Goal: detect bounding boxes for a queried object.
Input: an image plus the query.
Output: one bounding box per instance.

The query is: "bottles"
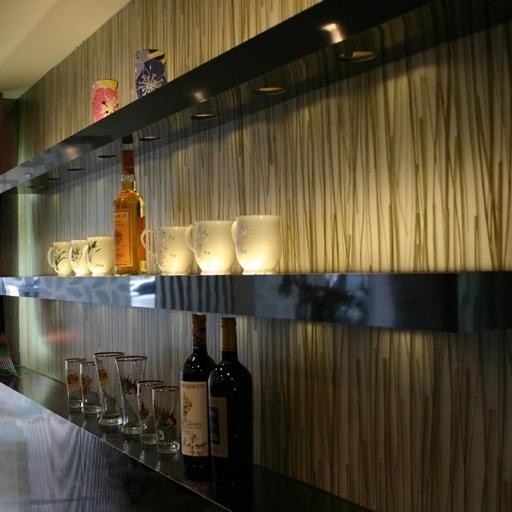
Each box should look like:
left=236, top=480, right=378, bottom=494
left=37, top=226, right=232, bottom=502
left=179, top=309, right=256, bottom=478
left=110, top=133, right=148, bottom=276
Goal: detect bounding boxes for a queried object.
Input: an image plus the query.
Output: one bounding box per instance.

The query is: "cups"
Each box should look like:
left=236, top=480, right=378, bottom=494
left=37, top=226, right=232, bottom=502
left=62, top=349, right=178, bottom=457
left=139, top=215, right=286, bottom=276
left=90, top=47, right=168, bottom=127
left=45, top=235, right=114, bottom=279
